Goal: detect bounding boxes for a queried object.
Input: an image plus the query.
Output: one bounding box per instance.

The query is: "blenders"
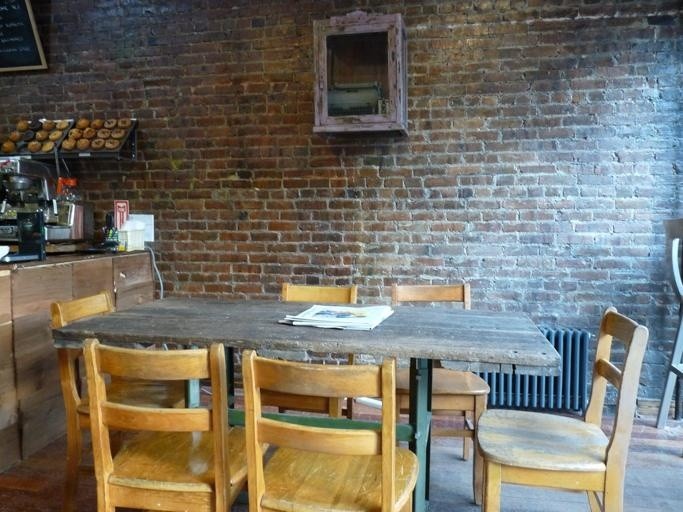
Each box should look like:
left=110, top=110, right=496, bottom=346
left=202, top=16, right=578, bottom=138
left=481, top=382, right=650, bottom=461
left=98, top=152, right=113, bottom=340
left=44, top=202, right=96, bottom=243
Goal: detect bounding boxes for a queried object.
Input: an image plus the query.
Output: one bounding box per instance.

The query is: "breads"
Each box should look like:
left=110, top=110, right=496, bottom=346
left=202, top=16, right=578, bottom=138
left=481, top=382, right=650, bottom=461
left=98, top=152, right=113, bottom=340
left=2, top=117, right=132, bottom=153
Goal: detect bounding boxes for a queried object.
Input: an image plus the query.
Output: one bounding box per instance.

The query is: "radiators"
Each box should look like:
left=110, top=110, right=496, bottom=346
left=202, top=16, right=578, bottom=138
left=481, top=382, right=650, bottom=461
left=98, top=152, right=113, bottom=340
left=476, top=324, right=590, bottom=413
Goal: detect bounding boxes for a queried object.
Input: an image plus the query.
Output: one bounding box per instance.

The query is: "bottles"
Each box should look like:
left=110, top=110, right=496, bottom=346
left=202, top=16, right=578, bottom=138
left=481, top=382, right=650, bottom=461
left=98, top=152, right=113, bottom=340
left=103, top=211, right=115, bottom=244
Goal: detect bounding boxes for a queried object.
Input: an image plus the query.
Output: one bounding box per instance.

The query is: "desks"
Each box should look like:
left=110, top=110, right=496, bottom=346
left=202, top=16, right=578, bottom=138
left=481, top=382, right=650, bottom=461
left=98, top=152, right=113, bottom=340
left=51, top=294, right=562, bottom=511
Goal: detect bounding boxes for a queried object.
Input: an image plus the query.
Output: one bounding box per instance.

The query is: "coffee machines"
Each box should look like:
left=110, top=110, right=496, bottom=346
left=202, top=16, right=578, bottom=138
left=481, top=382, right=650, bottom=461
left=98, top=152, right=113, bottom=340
left=0, top=157, right=54, bottom=262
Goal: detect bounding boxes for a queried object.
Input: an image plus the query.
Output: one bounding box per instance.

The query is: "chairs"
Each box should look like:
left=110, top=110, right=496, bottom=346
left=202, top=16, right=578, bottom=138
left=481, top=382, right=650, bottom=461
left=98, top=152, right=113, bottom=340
left=82, top=336, right=270, bottom=511
left=50, top=291, right=187, bottom=511
left=476, top=305, right=650, bottom=512
left=391, top=282, right=490, bottom=508
left=259, top=281, right=360, bottom=420
left=239, top=346, right=419, bottom=511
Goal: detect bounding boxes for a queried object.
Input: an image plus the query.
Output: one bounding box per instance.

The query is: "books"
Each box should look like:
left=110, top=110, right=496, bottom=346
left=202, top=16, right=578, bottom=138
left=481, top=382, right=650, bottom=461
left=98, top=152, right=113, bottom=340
left=277, top=304, right=395, bottom=331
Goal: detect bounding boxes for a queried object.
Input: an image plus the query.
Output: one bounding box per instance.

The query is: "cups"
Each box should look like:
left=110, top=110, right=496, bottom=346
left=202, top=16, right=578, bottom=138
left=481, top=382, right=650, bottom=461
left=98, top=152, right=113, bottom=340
left=114, top=230, right=127, bottom=253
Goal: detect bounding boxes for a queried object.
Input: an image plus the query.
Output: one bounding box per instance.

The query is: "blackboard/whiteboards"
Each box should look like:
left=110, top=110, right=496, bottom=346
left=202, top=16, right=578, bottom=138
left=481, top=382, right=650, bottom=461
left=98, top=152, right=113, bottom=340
left=0, top=0, right=49, bottom=72
